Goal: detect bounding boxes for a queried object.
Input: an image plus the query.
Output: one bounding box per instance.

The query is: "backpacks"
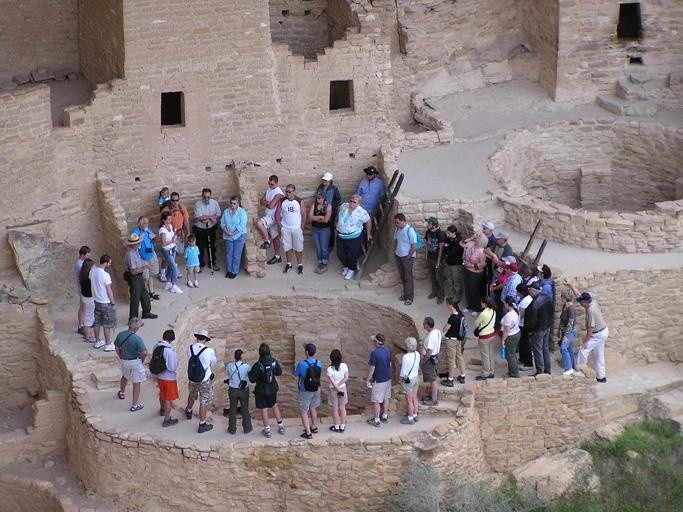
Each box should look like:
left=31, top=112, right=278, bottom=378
left=188, top=344, right=211, bottom=382
left=149, top=346, right=170, bottom=374
left=302, top=360, right=321, bottom=391
left=257, top=359, right=277, bottom=384
left=459, top=317, right=469, bottom=339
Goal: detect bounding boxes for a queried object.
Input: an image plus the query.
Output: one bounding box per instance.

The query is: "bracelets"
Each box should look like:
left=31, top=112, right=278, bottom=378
left=111, top=304, right=115, bottom=306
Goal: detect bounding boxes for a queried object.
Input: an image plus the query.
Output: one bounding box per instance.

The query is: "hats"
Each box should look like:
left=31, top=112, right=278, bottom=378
left=364, top=166, right=379, bottom=175
left=425, top=217, right=438, bottom=225
left=502, top=256, right=591, bottom=302
left=371, top=334, right=385, bottom=345
left=321, top=172, right=333, bottom=181
left=194, top=330, right=211, bottom=341
left=313, top=191, right=327, bottom=198
left=482, top=222, right=494, bottom=230
left=302, top=343, right=316, bottom=353
left=124, top=233, right=144, bottom=245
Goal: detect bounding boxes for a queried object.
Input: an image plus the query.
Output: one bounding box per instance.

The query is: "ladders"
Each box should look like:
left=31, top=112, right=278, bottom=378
left=355, top=169, right=405, bottom=281
left=517, top=219, right=548, bottom=283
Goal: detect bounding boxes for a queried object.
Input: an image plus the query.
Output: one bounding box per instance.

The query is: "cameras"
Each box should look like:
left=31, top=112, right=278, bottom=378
left=224, top=378, right=229, bottom=385
left=239, top=380, right=247, bottom=388
left=404, top=376, right=410, bottom=383
left=146, top=248, right=152, bottom=253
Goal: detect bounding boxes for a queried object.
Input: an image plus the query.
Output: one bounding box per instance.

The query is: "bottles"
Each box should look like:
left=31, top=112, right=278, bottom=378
left=499, top=346, right=505, bottom=359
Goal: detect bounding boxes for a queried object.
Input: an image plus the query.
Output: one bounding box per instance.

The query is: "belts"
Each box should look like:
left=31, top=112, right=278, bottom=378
left=592, top=325, right=606, bottom=333
left=123, top=358, right=140, bottom=360
left=446, top=337, right=457, bottom=340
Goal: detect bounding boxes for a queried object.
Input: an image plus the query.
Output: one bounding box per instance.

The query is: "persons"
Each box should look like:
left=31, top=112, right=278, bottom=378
left=399, top=337, right=422, bottom=424
left=132, top=215, right=160, bottom=300
left=153, top=329, right=180, bottom=427
left=92, top=255, right=116, bottom=352
left=423, top=217, right=610, bottom=387
left=114, top=318, right=148, bottom=412
left=248, top=343, right=285, bottom=437
left=159, top=186, right=247, bottom=294
left=227, top=349, right=254, bottom=434
left=421, top=317, right=442, bottom=406
left=76, top=246, right=91, bottom=334
left=257, top=166, right=384, bottom=280
left=327, top=350, right=348, bottom=433
left=366, top=333, right=391, bottom=427
left=186, top=330, right=217, bottom=433
left=79, top=259, right=96, bottom=343
left=294, top=344, right=322, bottom=439
left=393, top=213, right=417, bottom=305
left=125, top=232, right=158, bottom=327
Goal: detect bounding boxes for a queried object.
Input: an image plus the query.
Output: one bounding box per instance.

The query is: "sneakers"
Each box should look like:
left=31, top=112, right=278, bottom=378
left=261, top=240, right=355, bottom=280
left=399, top=293, right=478, bottom=316
left=148, top=263, right=236, bottom=301
left=78, top=312, right=157, bottom=351
left=160, top=406, right=344, bottom=438
left=441, top=359, right=606, bottom=387
left=368, top=397, right=438, bottom=427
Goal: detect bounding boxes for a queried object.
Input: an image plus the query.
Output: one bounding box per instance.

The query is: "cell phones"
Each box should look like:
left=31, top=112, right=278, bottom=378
left=337, top=392, right=344, bottom=396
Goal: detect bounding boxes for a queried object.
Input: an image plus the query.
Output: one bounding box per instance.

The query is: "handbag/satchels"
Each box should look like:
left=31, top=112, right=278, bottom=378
left=474, top=327, right=481, bottom=337
left=407, top=226, right=422, bottom=250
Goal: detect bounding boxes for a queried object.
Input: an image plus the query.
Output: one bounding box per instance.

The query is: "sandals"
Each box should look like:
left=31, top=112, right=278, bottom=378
left=118, top=391, right=125, bottom=399
left=131, top=403, right=144, bottom=410
